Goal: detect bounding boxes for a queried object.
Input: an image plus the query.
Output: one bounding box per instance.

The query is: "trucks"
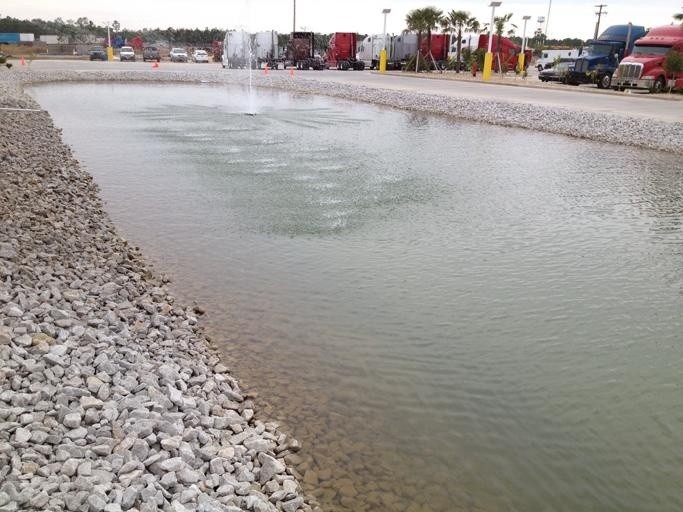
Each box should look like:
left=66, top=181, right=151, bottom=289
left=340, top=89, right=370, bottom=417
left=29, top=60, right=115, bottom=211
left=610, top=24, right=683, bottom=95
left=534, top=48, right=587, bottom=73
left=219, top=27, right=532, bottom=72
left=564, top=23, right=645, bottom=88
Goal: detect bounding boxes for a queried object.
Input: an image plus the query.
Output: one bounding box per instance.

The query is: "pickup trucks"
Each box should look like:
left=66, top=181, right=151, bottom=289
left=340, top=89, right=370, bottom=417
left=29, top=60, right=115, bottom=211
left=143, top=47, right=161, bottom=62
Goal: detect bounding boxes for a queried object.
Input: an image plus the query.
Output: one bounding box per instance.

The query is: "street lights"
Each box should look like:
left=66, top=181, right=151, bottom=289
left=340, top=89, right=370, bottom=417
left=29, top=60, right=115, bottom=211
left=536, top=16, right=545, bottom=29
left=106, top=19, right=114, bottom=60
left=516, top=16, right=530, bottom=75
left=482, top=1, right=502, bottom=80
left=378, top=8, right=391, bottom=71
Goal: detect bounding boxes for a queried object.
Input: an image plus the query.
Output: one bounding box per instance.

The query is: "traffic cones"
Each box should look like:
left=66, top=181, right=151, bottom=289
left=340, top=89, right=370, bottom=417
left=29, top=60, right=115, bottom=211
left=264, top=64, right=268, bottom=74
left=152, top=63, right=159, bottom=69
left=290, top=65, right=295, bottom=75
left=20, top=55, right=26, bottom=65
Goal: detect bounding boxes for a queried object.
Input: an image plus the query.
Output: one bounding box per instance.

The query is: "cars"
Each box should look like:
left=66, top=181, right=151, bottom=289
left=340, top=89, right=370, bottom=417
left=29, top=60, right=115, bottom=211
left=89, top=46, right=106, bottom=61
left=170, top=48, right=188, bottom=64
left=539, top=63, right=573, bottom=83
left=190, top=50, right=211, bottom=64
left=119, top=46, right=135, bottom=62
